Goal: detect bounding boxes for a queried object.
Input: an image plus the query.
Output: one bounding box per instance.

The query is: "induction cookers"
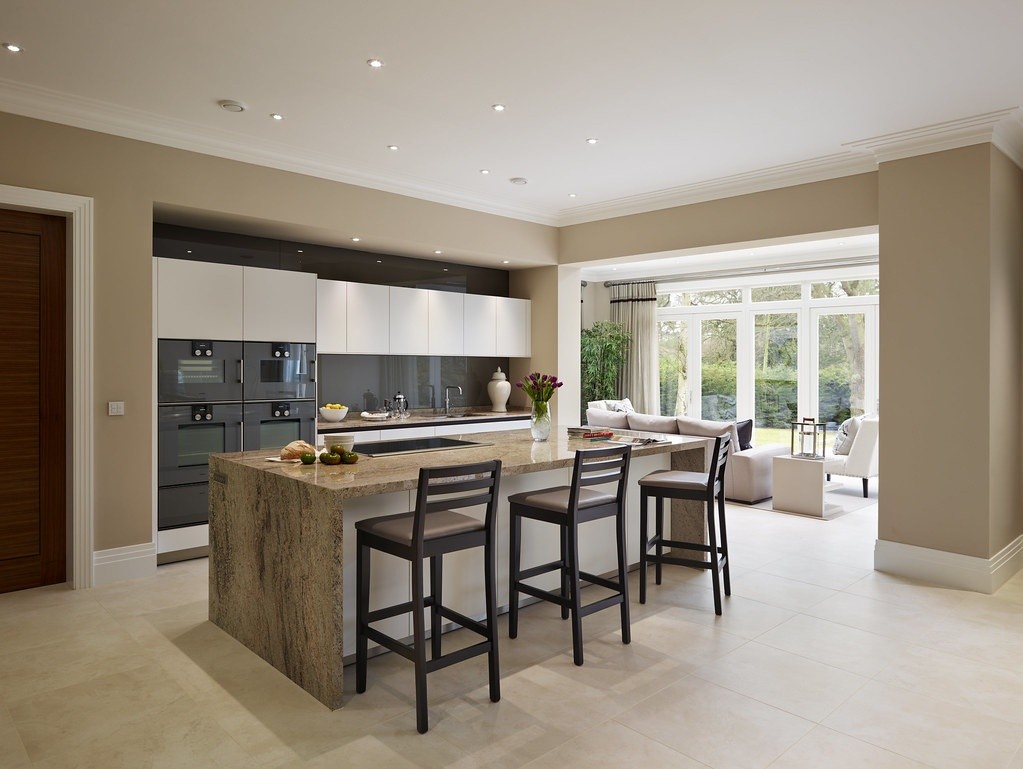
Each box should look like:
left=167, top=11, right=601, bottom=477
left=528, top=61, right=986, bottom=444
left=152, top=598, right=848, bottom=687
left=351, top=436, right=495, bottom=457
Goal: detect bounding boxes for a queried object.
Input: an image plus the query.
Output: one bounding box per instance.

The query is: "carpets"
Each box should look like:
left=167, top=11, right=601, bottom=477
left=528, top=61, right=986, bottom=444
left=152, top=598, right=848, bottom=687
left=726, top=479, right=878, bottom=521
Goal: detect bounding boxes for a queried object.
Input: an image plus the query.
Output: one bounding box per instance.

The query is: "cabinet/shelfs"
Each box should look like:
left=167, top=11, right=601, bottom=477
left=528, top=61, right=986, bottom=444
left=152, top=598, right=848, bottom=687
left=152, top=236, right=532, bottom=359
left=317, top=418, right=532, bottom=447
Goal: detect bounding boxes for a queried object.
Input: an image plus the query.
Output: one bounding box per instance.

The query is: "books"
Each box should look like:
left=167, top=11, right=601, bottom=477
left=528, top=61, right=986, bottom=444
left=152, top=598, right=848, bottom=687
left=567, top=427, right=666, bottom=446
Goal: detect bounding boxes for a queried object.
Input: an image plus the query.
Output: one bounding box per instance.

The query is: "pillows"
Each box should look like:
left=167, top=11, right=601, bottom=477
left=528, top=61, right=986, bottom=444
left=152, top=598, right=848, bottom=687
left=605, top=397, right=636, bottom=413
left=832, top=415, right=865, bottom=456
left=736, top=420, right=756, bottom=450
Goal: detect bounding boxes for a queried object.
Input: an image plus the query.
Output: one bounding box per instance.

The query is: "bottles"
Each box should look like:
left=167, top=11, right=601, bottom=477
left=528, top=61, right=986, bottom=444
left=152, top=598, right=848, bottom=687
left=487, top=367, right=512, bottom=412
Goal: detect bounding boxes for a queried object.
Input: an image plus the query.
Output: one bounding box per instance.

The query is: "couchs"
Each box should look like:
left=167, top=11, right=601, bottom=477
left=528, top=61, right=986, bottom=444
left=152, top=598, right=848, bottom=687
left=587, top=400, right=789, bottom=506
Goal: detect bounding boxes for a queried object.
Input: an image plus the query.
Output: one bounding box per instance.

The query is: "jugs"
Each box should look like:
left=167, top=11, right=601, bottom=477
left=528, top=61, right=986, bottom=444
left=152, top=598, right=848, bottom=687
left=360, top=389, right=378, bottom=410
left=392, top=391, right=409, bottom=412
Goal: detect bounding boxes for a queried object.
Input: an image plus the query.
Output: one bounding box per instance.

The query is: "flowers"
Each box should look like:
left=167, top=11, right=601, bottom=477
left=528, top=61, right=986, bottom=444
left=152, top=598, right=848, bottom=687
left=516, top=373, right=563, bottom=424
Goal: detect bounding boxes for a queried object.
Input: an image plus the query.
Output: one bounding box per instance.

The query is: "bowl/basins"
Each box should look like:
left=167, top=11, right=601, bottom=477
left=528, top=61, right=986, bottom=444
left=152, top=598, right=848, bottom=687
left=319, top=407, right=349, bottom=423
left=323, top=434, right=355, bottom=454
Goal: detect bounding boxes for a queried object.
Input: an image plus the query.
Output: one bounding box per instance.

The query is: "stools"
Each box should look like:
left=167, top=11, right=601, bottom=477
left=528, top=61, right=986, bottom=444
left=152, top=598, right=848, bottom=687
left=355, top=460, right=503, bottom=733
left=638, top=432, right=732, bottom=616
left=509, top=445, right=632, bottom=666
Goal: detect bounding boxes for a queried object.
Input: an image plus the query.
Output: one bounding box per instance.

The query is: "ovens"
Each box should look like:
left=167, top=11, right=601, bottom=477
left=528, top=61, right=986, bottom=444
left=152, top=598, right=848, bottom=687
left=156, top=335, right=318, bottom=530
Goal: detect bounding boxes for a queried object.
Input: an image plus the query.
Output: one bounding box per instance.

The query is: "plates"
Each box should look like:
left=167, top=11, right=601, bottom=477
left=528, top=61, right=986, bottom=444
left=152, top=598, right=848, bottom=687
left=363, top=415, right=388, bottom=421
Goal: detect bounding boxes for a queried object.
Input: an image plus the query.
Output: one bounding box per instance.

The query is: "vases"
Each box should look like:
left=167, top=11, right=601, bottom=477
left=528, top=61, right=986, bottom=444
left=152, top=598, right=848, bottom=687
left=530, top=401, right=551, bottom=441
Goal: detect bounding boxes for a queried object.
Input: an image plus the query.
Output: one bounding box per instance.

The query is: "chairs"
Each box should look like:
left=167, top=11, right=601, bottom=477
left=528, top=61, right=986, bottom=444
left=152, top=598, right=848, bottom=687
left=823, top=415, right=880, bottom=498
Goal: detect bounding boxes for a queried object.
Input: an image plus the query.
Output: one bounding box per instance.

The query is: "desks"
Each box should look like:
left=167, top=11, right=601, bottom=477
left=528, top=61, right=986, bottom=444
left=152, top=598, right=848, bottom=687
left=790, top=421, right=826, bottom=455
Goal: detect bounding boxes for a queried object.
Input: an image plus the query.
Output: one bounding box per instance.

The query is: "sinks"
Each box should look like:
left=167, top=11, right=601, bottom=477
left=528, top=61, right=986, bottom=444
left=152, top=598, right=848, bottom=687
left=420, top=413, right=494, bottom=418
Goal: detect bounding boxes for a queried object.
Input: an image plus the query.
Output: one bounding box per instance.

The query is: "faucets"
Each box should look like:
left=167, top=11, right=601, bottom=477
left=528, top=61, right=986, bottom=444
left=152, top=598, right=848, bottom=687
left=418, top=384, right=435, bottom=408
left=443, top=385, right=462, bottom=414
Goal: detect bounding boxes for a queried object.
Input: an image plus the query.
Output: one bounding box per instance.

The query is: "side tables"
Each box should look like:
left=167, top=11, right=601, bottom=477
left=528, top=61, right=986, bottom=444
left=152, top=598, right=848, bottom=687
left=772, top=454, right=845, bottom=518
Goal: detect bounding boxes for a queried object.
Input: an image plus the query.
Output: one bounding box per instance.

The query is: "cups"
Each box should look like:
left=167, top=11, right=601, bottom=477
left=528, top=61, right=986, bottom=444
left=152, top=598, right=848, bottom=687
left=391, top=410, right=410, bottom=419
